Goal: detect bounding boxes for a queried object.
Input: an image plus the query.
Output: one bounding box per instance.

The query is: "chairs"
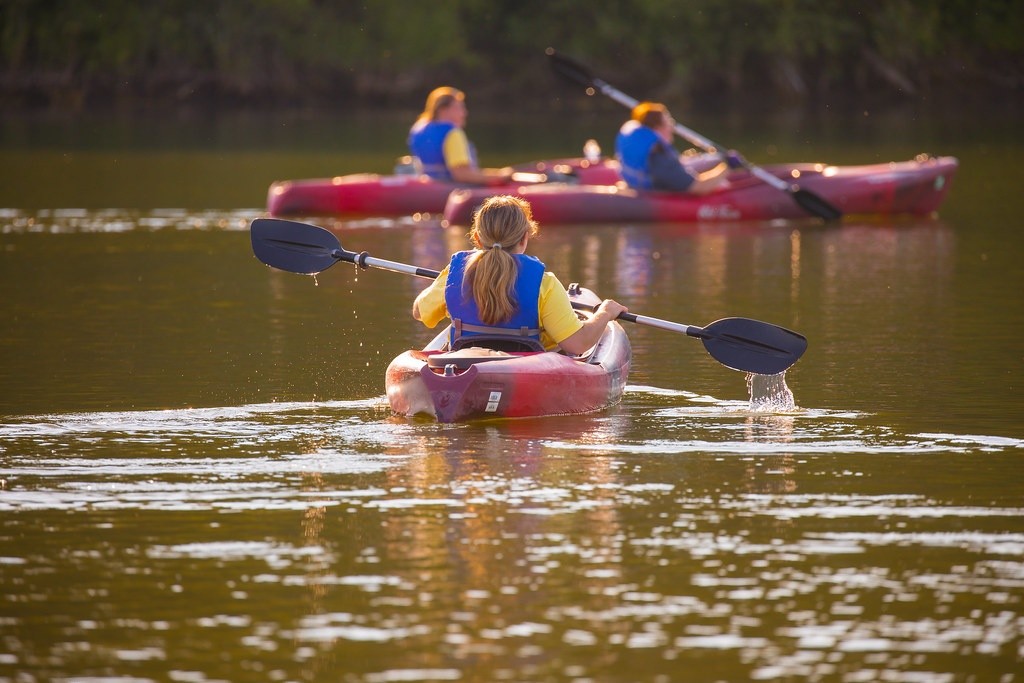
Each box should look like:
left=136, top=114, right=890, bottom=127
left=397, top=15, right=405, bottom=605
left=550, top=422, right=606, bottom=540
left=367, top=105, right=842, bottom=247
left=451, top=334, right=544, bottom=352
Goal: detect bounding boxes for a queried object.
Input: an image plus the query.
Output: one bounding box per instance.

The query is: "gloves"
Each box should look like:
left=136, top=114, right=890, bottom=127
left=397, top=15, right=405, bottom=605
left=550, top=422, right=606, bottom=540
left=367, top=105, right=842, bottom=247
left=722, top=150, right=743, bottom=169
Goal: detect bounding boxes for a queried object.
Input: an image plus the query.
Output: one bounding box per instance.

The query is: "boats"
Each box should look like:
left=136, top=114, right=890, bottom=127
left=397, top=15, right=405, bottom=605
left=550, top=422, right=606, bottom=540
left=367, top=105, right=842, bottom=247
left=385, top=281, right=633, bottom=424
left=442, top=155, right=957, bottom=226
left=269, top=152, right=720, bottom=239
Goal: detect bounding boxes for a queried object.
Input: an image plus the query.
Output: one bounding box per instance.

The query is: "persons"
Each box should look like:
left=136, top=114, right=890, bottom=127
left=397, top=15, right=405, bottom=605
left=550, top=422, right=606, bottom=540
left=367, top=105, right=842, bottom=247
left=406, top=88, right=515, bottom=188
left=411, top=195, right=629, bottom=356
left=617, top=102, right=745, bottom=198
